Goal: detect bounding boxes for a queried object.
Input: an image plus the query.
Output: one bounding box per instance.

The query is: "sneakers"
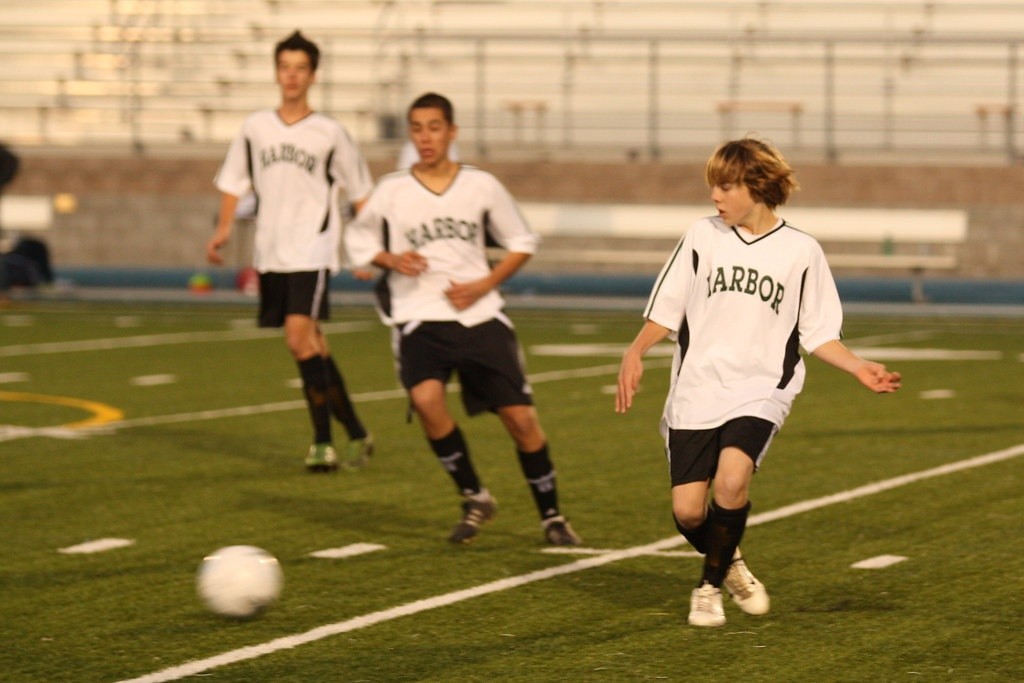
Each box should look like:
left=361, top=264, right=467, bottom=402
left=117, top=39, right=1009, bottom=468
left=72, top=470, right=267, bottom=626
left=722, top=547, right=772, bottom=615
left=452, top=495, right=498, bottom=543
left=543, top=516, right=584, bottom=546
left=687, top=583, right=727, bottom=627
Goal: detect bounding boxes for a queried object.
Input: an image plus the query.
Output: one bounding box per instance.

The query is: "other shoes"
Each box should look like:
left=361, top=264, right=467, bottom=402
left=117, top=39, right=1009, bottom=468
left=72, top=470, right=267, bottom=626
left=343, top=434, right=374, bottom=474
left=307, top=443, right=337, bottom=471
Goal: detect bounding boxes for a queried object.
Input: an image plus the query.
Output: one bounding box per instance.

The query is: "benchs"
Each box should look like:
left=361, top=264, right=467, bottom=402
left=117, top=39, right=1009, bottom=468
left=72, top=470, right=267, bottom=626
left=507, top=198, right=970, bottom=310
left=0, top=0, right=1024, bottom=166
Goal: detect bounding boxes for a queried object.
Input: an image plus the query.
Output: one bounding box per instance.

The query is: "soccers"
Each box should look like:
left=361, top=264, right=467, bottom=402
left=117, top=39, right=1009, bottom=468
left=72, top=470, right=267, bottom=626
left=196, top=543, right=286, bottom=620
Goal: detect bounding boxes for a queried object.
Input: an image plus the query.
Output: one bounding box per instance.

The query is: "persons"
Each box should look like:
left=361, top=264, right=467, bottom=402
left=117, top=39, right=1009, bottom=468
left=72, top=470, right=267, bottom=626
left=0, top=146, right=78, bottom=295
left=208, top=32, right=373, bottom=470
left=614, top=137, right=903, bottom=627
left=344, top=93, right=583, bottom=548
